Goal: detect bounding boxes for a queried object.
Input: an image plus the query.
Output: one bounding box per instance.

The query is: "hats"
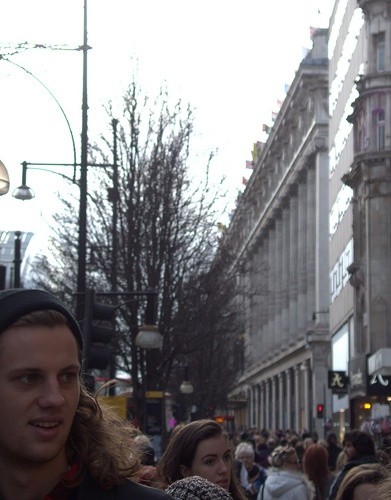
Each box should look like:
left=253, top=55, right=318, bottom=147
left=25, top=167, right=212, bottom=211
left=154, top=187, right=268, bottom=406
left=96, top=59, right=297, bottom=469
left=0, top=289, right=85, bottom=350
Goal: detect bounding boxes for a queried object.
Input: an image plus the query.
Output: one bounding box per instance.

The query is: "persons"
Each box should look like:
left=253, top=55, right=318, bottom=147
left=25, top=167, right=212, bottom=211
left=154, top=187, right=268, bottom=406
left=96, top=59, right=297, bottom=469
left=158, top=420, right=244, bottom=500
left=0, top=288, right=170, bottom=500
left=232, top=427, right=391, bottom=500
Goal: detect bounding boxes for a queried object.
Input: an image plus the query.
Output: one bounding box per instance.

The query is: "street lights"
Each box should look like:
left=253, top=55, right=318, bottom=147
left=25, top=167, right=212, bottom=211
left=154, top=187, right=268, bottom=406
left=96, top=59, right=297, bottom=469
left=157, top=364, right=193, bottom=437
left=12, top=161, right=87, bottom=381
left=78, top=287, right=163, bottom=349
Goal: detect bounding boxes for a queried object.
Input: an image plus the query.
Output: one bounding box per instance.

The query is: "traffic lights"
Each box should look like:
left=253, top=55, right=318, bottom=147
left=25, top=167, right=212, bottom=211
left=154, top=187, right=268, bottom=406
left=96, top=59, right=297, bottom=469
left=82, top=290, right=115, bottom=370
left=317, top=403, right=323, bottom=419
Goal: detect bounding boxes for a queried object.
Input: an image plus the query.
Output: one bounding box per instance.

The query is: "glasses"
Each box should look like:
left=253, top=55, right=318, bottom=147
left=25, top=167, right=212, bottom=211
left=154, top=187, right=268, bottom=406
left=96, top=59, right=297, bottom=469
left=288, top=459, right=300, bottom=465
